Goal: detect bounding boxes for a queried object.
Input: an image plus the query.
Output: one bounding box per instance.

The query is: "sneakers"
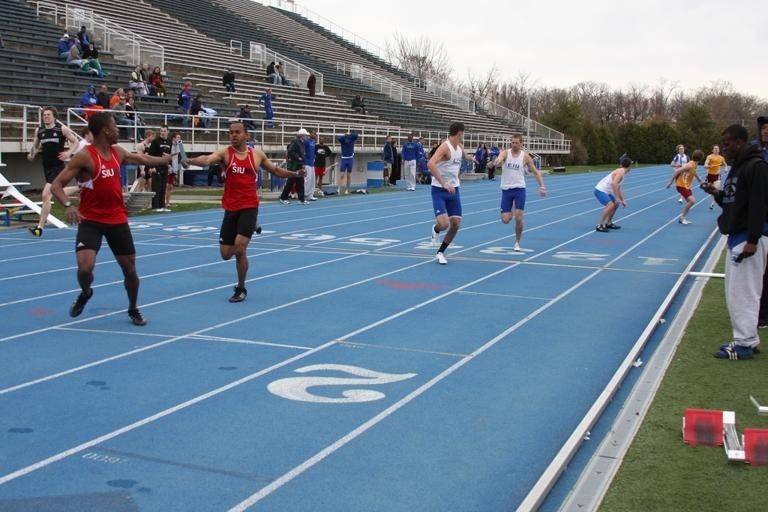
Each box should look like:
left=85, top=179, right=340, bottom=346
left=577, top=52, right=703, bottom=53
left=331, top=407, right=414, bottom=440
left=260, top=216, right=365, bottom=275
left=595, top=223, right=610, bottom=232
left=677, top=197, right=715, bottom=225
left=228, top=286, right=247, bottom=302
left=606, top=223, right=621, bottom=229
left=435, top=250, right=447, bottom=264
left=407, top=186, right=416, bottom=192
left=70, top=288, right=93, bottom=318
left=154, top=203, right=173, bottom=213
left=28, top=225, right=43, bottom=237
left=514, top=241, right=521, bottom=252
left=278, top=186, right=350, bottom=205
left=127, top=309, right=147, bottom=325
left=720, top=341, right=737, bottom=351
left=715, top=347, right=754, bottom=361
left=432, top=224, right=440, bottom=243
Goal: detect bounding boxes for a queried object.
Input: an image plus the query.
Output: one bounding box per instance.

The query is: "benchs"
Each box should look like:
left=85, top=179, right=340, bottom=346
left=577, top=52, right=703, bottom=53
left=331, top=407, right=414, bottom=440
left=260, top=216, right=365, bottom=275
left=0, top=0, right=532, bottom=148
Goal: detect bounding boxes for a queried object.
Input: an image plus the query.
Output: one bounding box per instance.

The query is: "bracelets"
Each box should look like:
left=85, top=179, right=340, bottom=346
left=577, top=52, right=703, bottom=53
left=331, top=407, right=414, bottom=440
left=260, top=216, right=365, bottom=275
left=540, top=187, right=544, bottom=190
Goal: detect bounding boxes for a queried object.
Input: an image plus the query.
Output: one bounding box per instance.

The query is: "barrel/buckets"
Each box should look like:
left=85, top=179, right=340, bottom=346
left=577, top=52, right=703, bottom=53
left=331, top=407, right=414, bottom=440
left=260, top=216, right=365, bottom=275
left=257, top=167, right=265, bottom=189
left=367, top=161, right=385, bottom=188
left=182, top=165, right=210, bottom=186
left=271, top=172, right=289, bottom=193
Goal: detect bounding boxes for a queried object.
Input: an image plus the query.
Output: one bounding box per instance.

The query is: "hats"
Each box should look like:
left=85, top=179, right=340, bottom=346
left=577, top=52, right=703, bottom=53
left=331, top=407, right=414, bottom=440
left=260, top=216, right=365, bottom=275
left=297, top=128, right=311, bottom=136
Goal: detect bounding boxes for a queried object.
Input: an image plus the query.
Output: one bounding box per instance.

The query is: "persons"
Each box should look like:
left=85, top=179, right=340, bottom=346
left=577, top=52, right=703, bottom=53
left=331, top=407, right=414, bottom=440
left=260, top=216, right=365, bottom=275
left=280, top=127, right=331, bottom=205
left=59, top=25, right=211, bottom=141
left=427, top=122, right=478, bottom=264
left=461, top=141, right=500, bottom=180
left=181, top=122, right=308, bottom=302
left=594, top=157, right=632, bottom=232
left=50, top=111, right=173, bottom=326
left=137, top=124, right=187, bottom=212
left=666, top=115, right=768, bottom=359
left=267, top=61, right=289, bottom=85
left=307, top=71, right=316, bottom=97
left=383, top=134, right=439, bottom=191
left=493, top=133, right=546, bottom=251
left=235, top=106, right=258, bottom=143
left=27, top=107, right=93, bottom=237
left=222, top=68, right=237, bottom=92
left=351, top=93, right=365, bottom=114
left=337, top=134, right=358, bottom=194
left=259, top=88, right=273, bottom=120
